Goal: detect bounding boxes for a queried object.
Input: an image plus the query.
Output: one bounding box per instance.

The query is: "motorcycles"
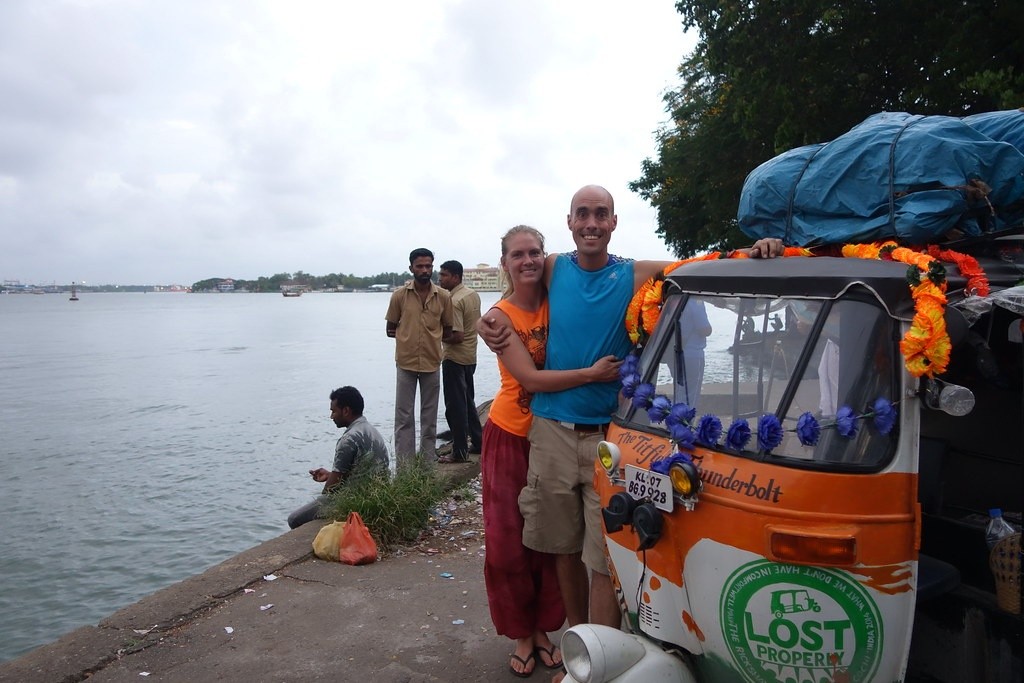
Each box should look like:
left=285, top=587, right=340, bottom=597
left=557, top=103, right=1024, bottom=683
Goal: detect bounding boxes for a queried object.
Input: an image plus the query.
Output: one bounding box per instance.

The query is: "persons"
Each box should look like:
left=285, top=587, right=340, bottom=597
left=768, top=314, right=783, bottom=332
left=480, top=225, right=625, bottom=678
left=660, top=295, right=712, bottom=431
left=476, top=184, right=783, bottom=683
left=287, top=385, right=389, bottom=530
left=742, top=316, right=755, bottom=333
left=437, top=260, right=483, bottom=461
left=790, top=300, right=839, bottom=417
left=385, top=247, right=454, bottom=476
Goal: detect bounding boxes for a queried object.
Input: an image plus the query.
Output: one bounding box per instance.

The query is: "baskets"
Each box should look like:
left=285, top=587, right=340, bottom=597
left=990, top=533, right=1021, bottom=614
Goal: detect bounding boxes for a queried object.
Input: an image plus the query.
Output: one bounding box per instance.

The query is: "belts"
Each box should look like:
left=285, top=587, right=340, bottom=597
left=548, top=418, right=608, bottom=433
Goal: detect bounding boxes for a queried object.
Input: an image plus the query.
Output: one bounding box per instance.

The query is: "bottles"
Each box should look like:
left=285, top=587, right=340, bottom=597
left=986, top=507, right=1013, bottom=553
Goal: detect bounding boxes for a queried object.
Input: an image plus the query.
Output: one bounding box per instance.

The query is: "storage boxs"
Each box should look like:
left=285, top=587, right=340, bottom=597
left=990, top=532, right=1024, bottom=614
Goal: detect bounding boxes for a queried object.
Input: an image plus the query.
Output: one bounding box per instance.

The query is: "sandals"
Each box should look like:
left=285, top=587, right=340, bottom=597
left=536, top=645, right=563, bottom=669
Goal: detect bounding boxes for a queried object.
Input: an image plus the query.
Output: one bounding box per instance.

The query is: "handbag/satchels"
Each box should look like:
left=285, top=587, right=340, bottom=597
left=312, top=509, right=378, bottom=564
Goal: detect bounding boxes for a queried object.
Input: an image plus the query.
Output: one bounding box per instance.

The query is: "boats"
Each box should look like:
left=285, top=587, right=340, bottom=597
left=282, top=290, right=302, bottom=297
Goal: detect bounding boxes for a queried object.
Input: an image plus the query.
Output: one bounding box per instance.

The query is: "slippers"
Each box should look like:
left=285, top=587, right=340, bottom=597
left=467, top=448, right=482, bottom=454
left=438, top=456, right=472, bottom=463
left=511, top=643, right=534, bottom=677
